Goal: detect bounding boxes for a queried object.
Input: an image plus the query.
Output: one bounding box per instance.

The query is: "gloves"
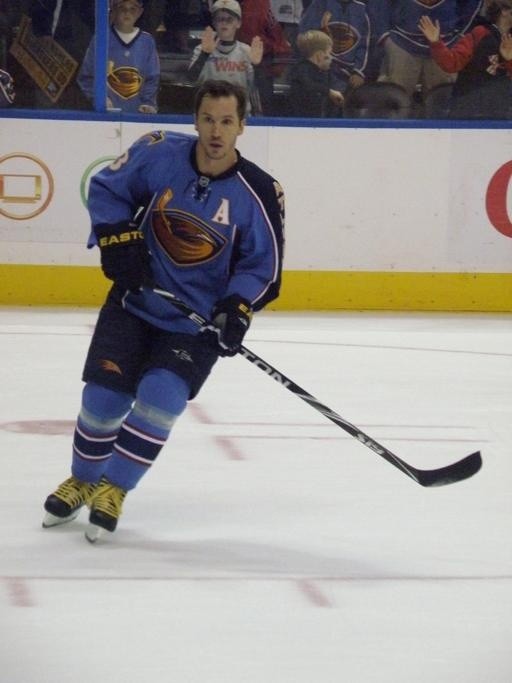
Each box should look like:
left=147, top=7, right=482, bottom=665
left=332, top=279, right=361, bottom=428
left=93, top=219, right=153, bottom=296
left=195, top=294, right=253, bottom=358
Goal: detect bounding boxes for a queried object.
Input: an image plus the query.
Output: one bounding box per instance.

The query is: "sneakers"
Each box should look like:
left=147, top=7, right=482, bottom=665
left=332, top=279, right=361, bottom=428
left=45, top=479, right=98, bottom=519
left=87, top=475, right=126, bottom=533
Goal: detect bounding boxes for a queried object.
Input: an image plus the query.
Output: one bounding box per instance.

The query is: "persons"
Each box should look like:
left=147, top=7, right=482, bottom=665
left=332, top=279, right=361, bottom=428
left=0, top=2, right=510, bottom=119
left=43, top=79, right=285, bottom=533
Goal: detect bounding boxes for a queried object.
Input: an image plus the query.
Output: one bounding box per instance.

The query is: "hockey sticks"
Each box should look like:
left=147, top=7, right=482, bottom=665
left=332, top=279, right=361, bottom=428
left=151, top=286, right=482, bottom=487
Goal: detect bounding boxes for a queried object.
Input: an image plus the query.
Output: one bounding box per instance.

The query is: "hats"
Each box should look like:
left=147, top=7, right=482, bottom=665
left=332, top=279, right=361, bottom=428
left=210, top=0, right=241, bottom=20
